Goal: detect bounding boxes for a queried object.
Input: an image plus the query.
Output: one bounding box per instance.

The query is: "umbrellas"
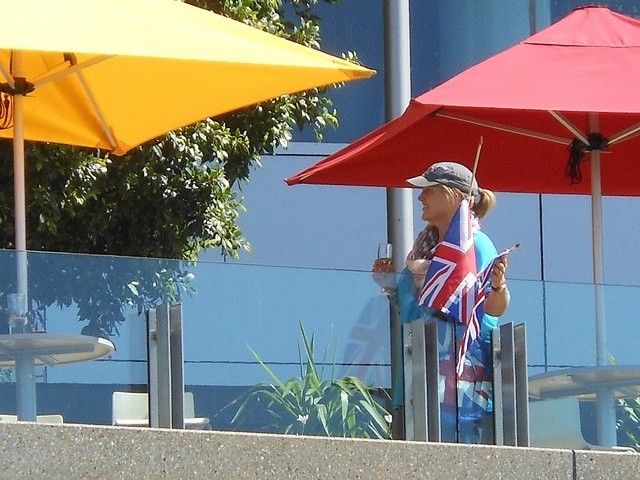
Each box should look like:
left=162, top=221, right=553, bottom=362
left=283, top=5, right=640, bottom=451
left=0, top=1, right=378, bottom=424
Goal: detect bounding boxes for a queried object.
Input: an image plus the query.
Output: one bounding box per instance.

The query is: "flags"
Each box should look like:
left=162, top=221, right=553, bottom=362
left=455, top=248, right=509, bottom=376
left=417, top=198, right=477, bottom=325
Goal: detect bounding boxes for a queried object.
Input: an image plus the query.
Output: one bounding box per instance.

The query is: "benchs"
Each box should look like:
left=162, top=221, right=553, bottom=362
left=112, top=391, right=213, bottom=430
left=528, top=398, right=637, bottom=453
left=0, top=415, right=64, bottom=423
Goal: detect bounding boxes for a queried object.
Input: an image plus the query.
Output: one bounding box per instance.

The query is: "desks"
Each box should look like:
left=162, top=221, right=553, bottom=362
left=528, top=365, right=640, bottom=401
left=0, top=333, right=114, bottom=422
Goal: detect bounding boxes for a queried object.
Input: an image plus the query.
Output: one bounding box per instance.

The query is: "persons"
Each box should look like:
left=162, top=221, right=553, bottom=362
left=371, top=161, right=510, bottom=443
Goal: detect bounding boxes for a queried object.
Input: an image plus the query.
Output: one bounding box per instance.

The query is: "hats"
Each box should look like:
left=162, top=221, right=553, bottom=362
left=407, top=162, right=479, bottom=196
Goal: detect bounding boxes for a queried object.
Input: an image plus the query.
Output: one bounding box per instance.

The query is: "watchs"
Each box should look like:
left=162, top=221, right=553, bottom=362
left=491, top=280, right=507, bottom=293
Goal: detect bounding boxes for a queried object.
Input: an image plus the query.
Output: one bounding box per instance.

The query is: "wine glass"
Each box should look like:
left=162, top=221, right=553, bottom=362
left=378, top=243, right=393, bottom=296
